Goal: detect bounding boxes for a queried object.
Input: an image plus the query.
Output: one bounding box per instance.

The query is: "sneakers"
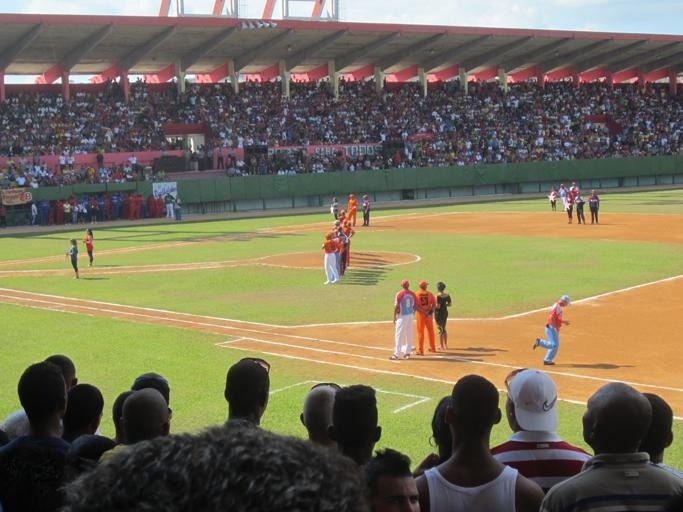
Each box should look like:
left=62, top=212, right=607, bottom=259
left=389, top=346, right=447, bottom=359
left=533, top=338, right=540, bottom=350
left=544, top=361, right=554, bottom=365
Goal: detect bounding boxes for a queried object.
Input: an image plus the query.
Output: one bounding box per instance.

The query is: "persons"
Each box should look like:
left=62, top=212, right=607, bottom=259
left=322, top=194, right=359, bottom=285
left=1, top=77, right=683, bottom=189
left=66, top=239, right=79, bottom=280
left=415, top=281, right=436, bottom=354
left=546, top=182, right=599, bottom=225
left=59, top=415, right=373, bottom=512
left=489, top=369, right=593, bottom=495
left=539, top=382, right=683, bottom=512
left=389, top=279, right=420, bottom=360
left=1, top=354, right=172, bottom=512
left=299, top=383, right=421, bottom=512
left=83, top=229, right=93, bottom=266
left=533, top=295, right=571, bottom=365
left=224, top=358, right=270, bottom=426
left=412, top=375, right=546, bottom=512
left=31, top=191, right=182, bottom=226
left=362, top=194, right=370, bottom=226
left=434, top=282, right=452, bottom=350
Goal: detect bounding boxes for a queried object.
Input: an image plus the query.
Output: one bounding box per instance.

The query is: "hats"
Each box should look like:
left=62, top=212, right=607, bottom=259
left=325, top=210, right=352, bottom=238
left=419, top=281, right=428, bottom=288
left=401, top=280, right=409, bottom=289
left=506, top=370, right=558, bottom=431
left=64, top=434, right=118, bottom=471
left=561, top=295, right=571, bottom=305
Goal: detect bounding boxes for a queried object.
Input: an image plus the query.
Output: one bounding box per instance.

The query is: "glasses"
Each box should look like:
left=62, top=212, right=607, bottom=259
left=311, top=384, right=342, bottom=391
left=504, top=368, right=530, bottom=395
left=241, top=358, right=269, bottom=373
